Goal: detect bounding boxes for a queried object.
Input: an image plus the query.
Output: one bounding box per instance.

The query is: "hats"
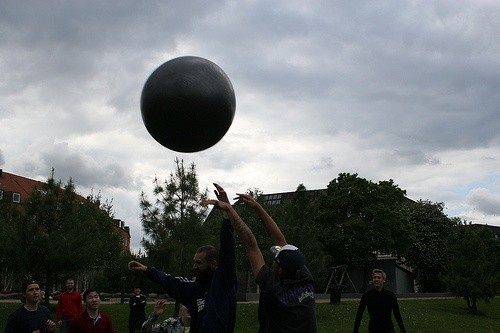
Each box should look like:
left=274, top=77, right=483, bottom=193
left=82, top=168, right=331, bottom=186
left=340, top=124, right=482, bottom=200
left=21, top=279, right=42, bottom=303
left=270, top=243, right=304, bottom=275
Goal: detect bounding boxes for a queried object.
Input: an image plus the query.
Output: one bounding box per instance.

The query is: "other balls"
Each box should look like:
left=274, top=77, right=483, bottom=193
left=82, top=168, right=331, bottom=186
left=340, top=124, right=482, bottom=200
left=139, top=56, right=236, bottom=154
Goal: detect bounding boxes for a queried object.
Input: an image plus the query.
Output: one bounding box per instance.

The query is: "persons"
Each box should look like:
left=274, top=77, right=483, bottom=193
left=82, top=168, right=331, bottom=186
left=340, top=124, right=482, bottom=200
left=69, top=288, right=115, bottom=333
left=4, top=279, right=56, bottom=333
left=128, top=183, right=236, bottom=333
left=129, top=285, right=191, bottom=333
left=201, top=193, right=318, bottom=333
left=56, top=278, right=83, bottom=333
left=353, top=269, right=406, bottom=333
